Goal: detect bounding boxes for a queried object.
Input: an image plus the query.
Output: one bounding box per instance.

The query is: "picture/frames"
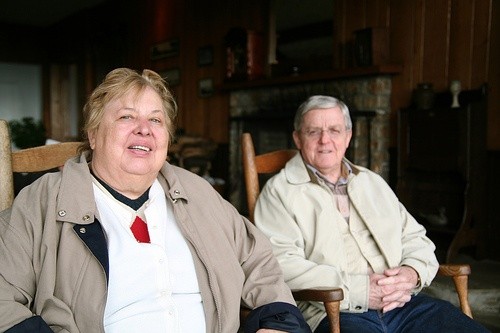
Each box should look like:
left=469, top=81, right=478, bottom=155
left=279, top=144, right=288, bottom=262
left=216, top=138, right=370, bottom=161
left=155, top=67, right=184, bottom=87
left=151, top=38, right=180, bottom=61
left=197, top=74, right=214, bottom=98
left=195, top=44, right=216, bottom=67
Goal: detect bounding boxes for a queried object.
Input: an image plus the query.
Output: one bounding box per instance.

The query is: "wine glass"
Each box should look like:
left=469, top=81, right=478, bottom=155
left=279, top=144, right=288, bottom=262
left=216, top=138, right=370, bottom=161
left=449, top=79, right=461, bottom=108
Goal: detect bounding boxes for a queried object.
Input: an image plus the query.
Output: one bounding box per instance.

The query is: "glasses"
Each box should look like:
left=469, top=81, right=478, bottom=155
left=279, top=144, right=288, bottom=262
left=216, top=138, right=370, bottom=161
left=301, top=126, right=349, bottom=138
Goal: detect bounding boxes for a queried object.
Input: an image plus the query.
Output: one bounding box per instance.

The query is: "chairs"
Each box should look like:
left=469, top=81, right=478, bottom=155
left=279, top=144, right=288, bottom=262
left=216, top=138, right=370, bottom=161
left=239, top=133, right=474, bottom=333
left=0, top=117, right=271, bottom=332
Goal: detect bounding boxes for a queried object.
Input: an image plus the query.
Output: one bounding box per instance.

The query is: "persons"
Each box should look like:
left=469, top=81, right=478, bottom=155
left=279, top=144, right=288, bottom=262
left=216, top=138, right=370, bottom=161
left=2, top=69, right=313, bottom=332
left=254, top=96, right=496, bottom=333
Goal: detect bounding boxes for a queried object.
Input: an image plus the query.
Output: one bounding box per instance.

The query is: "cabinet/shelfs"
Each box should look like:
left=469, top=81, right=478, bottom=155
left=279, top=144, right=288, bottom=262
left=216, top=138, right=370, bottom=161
left=394, top=84, right=491, bottom=262
